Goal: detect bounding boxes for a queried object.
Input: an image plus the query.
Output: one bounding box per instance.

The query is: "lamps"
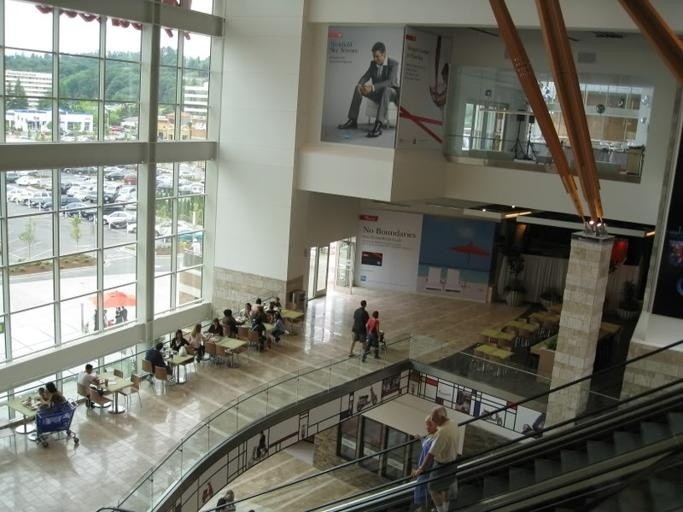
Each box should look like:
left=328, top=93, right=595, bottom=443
left=527, top=116, right=536, bottom=160
left=509, top=109, right=526, bottom=159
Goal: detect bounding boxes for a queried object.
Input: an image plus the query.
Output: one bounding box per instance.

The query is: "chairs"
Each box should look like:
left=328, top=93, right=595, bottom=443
left=424, top=267, right=443, bottom=294
left=365, top=65, right=400, bottom=131
left=444, top=268, right=463, bottom=297
left=77, top=301, right=305, bottom=417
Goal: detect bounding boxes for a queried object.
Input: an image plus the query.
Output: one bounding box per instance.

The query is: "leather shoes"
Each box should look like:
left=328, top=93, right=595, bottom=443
left=368, top=123, right=382, bottom=137
left=338, top=119, right=358, bottom=129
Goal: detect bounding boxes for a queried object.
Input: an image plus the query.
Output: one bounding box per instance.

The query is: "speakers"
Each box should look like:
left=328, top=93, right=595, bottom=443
left=529, top=116, right=534, bottom=123
left=516, top=109, right=526, bottom=121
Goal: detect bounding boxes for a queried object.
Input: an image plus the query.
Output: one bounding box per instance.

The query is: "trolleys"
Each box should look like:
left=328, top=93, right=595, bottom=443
left=34, top=398, right=80, bottom=447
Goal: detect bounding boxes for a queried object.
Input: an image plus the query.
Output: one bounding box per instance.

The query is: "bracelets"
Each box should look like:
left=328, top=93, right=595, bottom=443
left=418, top=465, right=424, bottom=470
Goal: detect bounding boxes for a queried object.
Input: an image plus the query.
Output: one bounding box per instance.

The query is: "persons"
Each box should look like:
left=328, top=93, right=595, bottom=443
left=222, top=309, right=245, bottom=334
left=413, top=404, right=459, bottom=512
left=237, top=296, right=286, bottom=352
left=206, top=481, right=213, bottom=497
left=361, top=310, right=383, bottom=362
left=337, top=40, right=401, bottom=138
left=38, top=388, right=50, bottom=401
left=169, top=329, right=190, bottom=352
left=121, top=306, right=128, bottom=322
left=144, top=342, right=167, bottom=384
left=189, top=323, right=212, bottom=363
left=411, top=414, right=439, bottom=512
left=78, top=364, right=99, bottom=408
left=115, top=307, right=122, bottom=324
left=37, top=382, right=66, bottom=408
left=254, top=430, right=269, bottom=459
left=214, top=498, right=226, bottom=511
left=348, top=299, right=371, bottom=357
left=222, top=490, right=236, bottom=511
left=208, top=318, right=223, bottom=337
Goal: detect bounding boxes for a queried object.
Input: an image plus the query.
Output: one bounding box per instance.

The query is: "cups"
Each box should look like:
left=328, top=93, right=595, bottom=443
left=105, top=378, right=109, bottom=387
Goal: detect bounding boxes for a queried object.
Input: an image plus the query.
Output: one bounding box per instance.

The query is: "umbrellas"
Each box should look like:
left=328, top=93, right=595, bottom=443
left=91, top=290, right=136, bottom=308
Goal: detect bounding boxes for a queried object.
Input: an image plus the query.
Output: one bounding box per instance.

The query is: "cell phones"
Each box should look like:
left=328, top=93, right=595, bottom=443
left=361, top=251, right=383, bottom=267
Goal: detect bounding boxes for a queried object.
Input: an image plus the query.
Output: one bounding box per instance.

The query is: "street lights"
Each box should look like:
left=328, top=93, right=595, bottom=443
left=107, top=105, right=111, bottom=126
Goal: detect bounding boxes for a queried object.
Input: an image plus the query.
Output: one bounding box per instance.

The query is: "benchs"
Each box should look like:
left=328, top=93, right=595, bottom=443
left=487, top=150, right=515, bottom=161
left=570, top=158, right=621, bottom=177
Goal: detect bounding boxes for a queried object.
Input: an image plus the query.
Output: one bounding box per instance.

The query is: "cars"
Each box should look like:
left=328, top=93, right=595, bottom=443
left=4, top=161, right=204, bottom=245
left=43, top=125, right=136, bottom=142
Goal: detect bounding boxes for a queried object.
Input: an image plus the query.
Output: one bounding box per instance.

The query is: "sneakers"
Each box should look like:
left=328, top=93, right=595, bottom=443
left=145, top=374, right=154, bottom=385
left=348, top=353, right=355, bottom=358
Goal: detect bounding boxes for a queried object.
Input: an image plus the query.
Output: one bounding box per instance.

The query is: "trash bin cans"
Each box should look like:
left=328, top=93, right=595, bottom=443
left=487, top=285, right=496, bottom=303
left=288, top=289, right=305, bottom=323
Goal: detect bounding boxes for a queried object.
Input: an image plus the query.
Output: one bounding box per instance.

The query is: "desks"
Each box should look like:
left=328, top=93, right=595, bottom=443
left=473, top=302, right=563, bottom=377
left=4, top=394, right=42, bottom=434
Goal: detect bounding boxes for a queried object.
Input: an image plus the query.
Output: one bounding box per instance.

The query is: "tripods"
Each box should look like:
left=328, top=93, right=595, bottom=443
left=510, top=123, right=526, bottom=158
left=526, top=125, right=536, bottom=158
